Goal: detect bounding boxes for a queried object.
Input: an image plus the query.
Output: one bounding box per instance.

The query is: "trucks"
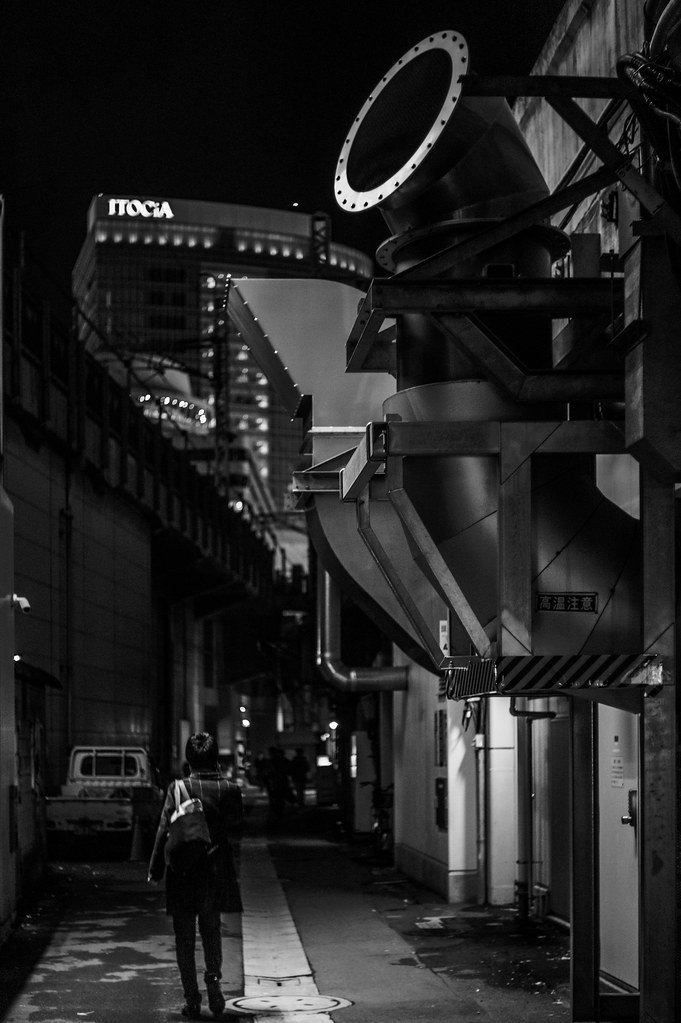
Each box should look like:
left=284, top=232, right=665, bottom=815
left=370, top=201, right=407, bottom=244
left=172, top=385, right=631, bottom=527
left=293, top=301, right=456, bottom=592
left=47, top=746, right=164, bottom=852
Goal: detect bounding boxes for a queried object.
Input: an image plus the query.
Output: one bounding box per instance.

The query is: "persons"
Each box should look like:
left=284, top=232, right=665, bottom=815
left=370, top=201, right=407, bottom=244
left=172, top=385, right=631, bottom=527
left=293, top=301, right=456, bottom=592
left=290, top=747, right=310, bottom=801
left=254, top=757, right=283, bottom=813
left=146, top=733, right=245, bottom=1019
left=274, top=748, right=290, bottom=803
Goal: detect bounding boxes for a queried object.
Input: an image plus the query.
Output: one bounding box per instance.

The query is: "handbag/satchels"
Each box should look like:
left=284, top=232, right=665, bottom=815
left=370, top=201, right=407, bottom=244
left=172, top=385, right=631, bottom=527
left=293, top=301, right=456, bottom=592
left=164, top=780, right=212, bottom=866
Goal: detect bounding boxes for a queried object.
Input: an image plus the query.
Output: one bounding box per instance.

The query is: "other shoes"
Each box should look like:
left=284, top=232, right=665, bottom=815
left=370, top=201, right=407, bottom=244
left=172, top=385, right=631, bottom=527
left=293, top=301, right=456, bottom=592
left=207, top=977, right=225, bottom=1013
left=183, top=1003, right=201, bottom=1019
